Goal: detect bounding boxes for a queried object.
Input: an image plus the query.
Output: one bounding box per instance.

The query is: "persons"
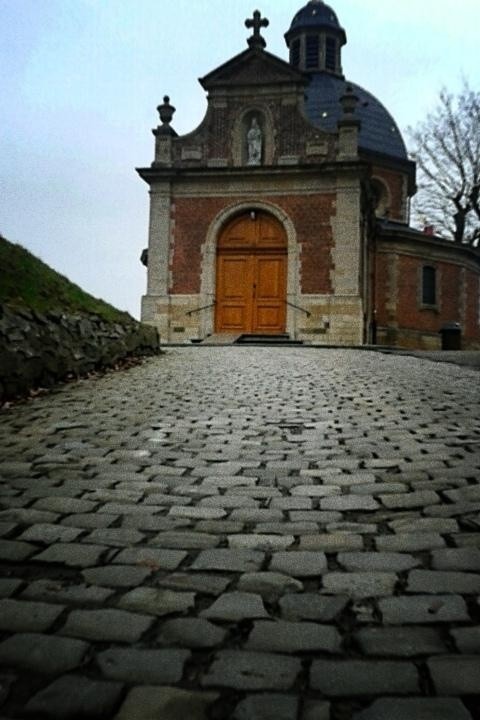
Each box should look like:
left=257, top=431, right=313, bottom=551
left=247, top=118, right=261, bottom=162
